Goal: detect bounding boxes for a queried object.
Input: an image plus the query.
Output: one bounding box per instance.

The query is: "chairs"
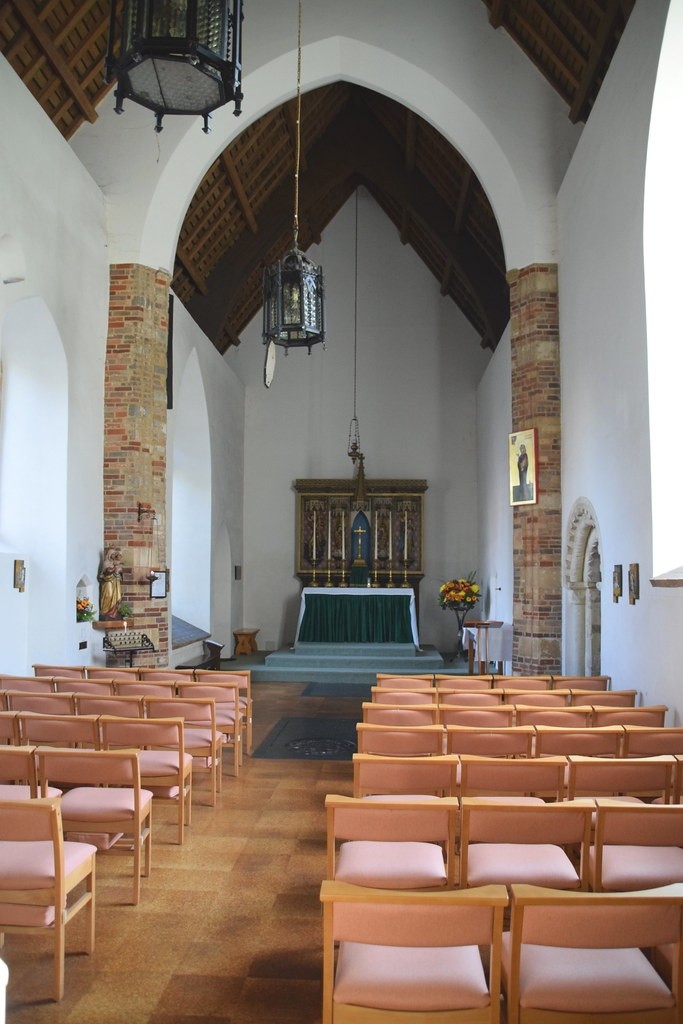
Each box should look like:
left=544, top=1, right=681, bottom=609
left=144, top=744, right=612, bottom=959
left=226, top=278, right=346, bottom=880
left=0, top=664, right=253, bottom=1000
left=320, top=673, right=683, bottom=1024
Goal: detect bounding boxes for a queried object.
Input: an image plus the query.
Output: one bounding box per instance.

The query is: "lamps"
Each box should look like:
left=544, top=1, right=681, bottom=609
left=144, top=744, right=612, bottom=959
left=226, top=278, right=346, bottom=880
left=104, top=0, right=244, bottom=134
left=263, top=0, right=327, bottom=356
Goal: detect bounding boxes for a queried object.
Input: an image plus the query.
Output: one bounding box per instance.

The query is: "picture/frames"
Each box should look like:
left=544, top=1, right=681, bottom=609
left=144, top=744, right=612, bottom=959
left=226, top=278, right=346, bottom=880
left=150, top=571, right=166, bottom=598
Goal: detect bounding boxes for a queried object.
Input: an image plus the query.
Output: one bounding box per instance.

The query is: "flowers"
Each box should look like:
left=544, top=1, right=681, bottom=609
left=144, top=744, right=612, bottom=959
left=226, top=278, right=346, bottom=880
left=77, top=596, right=97, bottom=621
left=438, top=571, right=482, bottom=611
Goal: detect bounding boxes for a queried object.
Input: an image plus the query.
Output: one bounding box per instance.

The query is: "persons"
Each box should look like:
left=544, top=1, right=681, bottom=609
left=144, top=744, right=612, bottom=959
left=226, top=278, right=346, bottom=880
left=98, top=549, right=123, bottom=621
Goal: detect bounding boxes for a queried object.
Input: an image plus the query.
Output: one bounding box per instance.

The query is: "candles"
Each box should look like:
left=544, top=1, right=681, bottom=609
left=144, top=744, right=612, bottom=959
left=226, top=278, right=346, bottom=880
left=313, top=511, right=316, bottom=560
left=389, top=512, right=392, bottom=560
left=375, top=511, right=378, bottom=560
left=328, top=511, right=331, bottom=560
left=404, top=511, right=407, bottom=559
left=342, top=511, right=345, bottom=560
